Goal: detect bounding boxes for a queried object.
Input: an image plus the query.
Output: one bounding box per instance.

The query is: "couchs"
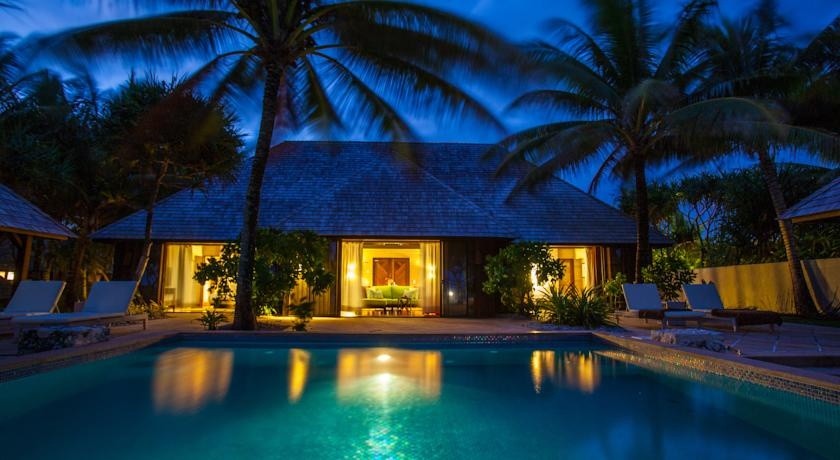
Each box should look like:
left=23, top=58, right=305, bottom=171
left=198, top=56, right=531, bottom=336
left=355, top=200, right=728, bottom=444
left=362, top=285, right=420, bottom=314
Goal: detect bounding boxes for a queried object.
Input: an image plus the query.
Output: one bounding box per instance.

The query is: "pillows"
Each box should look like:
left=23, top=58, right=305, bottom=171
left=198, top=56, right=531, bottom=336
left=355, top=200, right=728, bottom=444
left=366, top=288, right=383, bottom=298
left=403, top=289, right=415, bottom=299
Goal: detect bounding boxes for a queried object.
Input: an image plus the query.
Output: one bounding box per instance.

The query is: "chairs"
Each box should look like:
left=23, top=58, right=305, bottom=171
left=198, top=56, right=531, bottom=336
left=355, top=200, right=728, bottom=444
left=0, top=281, right=148, bottom=344
left=622, top=284, right=783, bottom=333
